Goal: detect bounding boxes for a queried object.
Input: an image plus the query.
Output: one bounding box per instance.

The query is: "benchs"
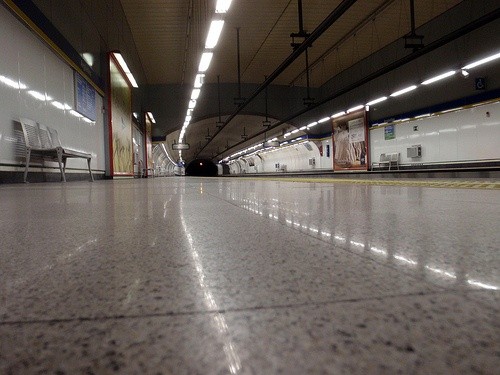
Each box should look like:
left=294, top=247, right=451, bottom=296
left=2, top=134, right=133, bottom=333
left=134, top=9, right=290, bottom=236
left=19, top=117, right=97, bottom=184
left=371, top=152, right=400, bottom=172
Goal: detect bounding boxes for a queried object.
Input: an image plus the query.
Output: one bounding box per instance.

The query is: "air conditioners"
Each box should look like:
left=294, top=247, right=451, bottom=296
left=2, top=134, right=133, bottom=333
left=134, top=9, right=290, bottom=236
left=309, top=157, right=316, bottom=166
left=407, top=145, right=421, bottom=158
